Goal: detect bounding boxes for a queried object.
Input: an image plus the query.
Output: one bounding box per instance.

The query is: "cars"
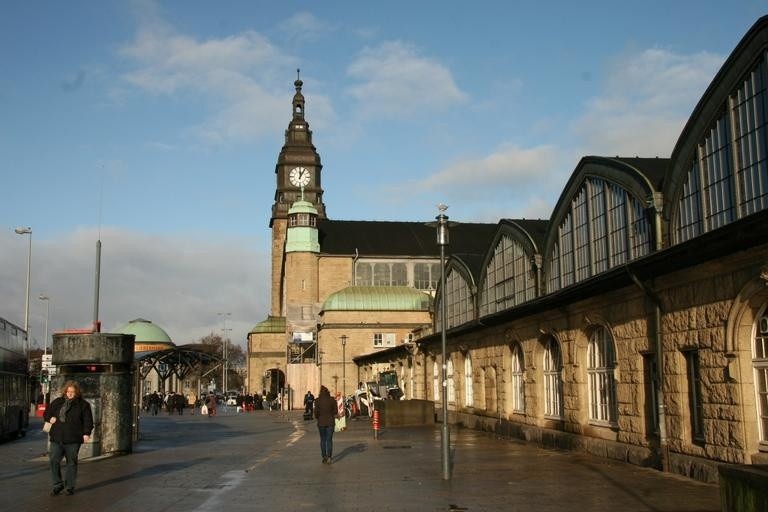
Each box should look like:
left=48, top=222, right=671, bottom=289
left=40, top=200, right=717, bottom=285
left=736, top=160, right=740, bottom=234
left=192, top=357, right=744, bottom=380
left=226, top=396, right=237, bottom=406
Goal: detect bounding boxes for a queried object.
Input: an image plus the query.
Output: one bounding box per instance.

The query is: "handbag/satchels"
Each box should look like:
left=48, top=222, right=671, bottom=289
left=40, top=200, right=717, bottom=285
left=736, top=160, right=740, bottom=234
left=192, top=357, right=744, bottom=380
left=206, top=396, right=210, bottom=404
left=43, top=422, right=52, bottom=433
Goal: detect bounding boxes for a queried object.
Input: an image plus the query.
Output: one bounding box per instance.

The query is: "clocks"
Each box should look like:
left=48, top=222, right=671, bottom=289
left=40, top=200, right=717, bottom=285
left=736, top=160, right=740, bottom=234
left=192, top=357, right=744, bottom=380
left=288, top=167, right=311, bottom=188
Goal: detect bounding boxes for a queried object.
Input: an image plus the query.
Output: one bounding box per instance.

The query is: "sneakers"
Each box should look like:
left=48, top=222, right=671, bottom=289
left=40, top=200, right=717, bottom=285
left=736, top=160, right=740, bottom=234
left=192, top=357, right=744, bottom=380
left=64, top=488, right=73, bottom=495
left=322, top=457, right=331, bottom=463
left=50, top=485, right=64, bottom=495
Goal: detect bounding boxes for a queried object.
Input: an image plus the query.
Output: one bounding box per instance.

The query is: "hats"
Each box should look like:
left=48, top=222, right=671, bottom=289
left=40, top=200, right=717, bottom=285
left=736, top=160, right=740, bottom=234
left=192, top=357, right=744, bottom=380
left=321, top=385, right=328, bottom=393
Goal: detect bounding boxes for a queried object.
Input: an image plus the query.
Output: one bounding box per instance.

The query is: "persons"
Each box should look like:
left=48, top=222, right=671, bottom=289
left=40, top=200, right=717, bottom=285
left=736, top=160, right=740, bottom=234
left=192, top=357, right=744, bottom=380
left=314, top=386, right=338, bottom=464
left=303, top=391, right=314, bottom=420
left=43, top=382, right=94, bottom=496
left=193, top=393, right=217, bottom=418
left=236, top=391, right=281, bottom=412
left=142, top=392, right=186, bottom=416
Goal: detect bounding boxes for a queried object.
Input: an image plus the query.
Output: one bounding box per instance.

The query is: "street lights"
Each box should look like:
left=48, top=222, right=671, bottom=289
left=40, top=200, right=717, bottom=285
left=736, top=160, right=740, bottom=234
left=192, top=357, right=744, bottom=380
left=427, top=203, right=463, bottom=483
left=39, top=293, right=49, bottom=354
left=338, top=335, right=349, bottom=397
left=14, top=227, right=32, bottom=330
